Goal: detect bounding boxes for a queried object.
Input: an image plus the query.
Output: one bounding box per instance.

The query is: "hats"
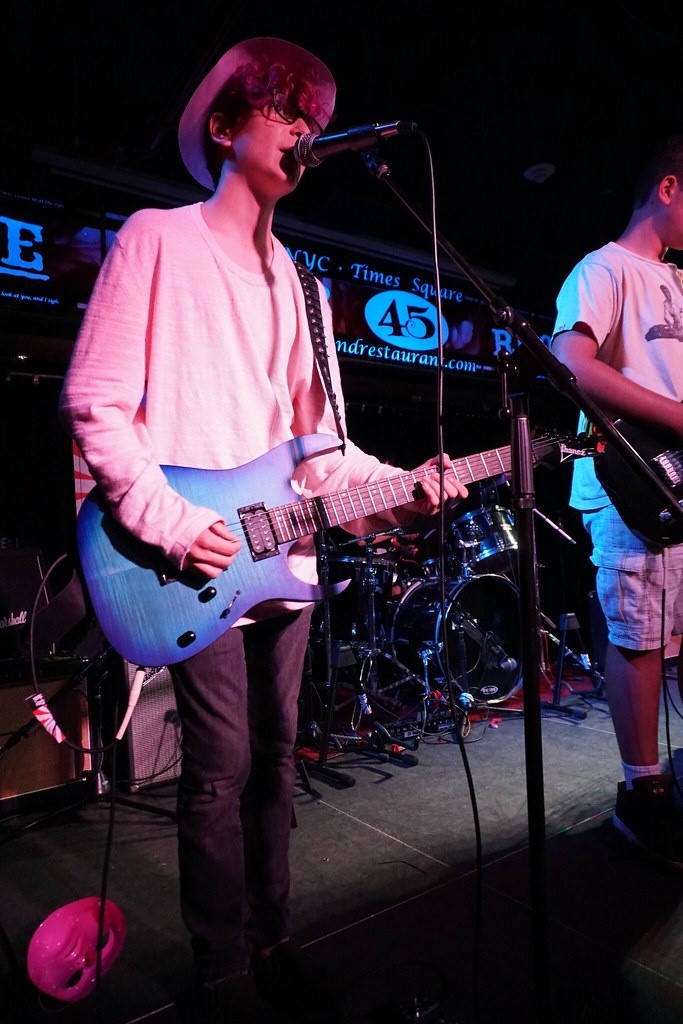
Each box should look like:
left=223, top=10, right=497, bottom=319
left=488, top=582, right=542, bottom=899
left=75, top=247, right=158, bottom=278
left=178, top=35, right=336, bottom=191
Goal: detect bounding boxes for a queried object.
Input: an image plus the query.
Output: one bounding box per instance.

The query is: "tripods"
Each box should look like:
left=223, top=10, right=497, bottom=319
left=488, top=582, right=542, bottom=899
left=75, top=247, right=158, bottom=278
left=0, top=648, right=176, bottom=845
left=527, top=511, right=608, bottom=701
left=291, top=528, right=523, bottom=761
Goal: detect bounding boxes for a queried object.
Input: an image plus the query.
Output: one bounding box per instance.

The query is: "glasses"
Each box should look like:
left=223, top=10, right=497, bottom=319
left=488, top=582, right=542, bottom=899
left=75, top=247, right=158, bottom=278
left=249, top=85, right=324, bottom=142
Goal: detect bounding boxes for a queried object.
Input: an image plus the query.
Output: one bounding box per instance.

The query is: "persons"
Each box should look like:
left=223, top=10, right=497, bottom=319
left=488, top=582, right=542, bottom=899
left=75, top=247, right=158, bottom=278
left=550, top=150, right=683, bottom=869
left=61, top=38, right=468, bottom=1024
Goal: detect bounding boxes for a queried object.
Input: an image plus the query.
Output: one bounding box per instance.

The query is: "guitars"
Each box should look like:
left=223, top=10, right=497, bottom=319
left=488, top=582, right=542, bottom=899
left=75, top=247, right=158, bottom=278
left=593, top=414, right=683, bottom=550
left=74, top=431, right=601, bottom=668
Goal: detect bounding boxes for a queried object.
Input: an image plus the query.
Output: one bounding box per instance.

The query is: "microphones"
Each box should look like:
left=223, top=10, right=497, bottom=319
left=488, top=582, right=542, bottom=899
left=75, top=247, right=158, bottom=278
left=486, top=636, right=517, bottom=671
left=293, top=120, right=416, bottom=168
left=0, top=726, right=29, bottom=760
left=169, top=712, right=179, bottom=723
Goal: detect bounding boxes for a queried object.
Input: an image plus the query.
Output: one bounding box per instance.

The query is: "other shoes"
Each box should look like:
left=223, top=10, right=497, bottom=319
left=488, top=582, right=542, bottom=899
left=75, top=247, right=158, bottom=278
left=198, top=962, right=268, bottom=1024
left=248, top=933, right=347, bottom=1009
left=612, top=773, right=683, bottom=862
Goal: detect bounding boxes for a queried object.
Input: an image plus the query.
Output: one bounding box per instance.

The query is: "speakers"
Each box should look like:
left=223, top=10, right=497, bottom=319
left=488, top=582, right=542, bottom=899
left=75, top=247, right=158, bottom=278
left=0, top=654, right=93, bottom=811
left=99, top=638, right=180, bottom=792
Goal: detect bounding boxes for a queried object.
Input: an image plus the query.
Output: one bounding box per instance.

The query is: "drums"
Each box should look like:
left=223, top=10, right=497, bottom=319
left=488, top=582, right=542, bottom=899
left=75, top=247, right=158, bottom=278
left=315, top=555, right=399, bottom=651
left=390, top=572, right=524, bottom=706
left=444, top=504, right=520, bottom=576
